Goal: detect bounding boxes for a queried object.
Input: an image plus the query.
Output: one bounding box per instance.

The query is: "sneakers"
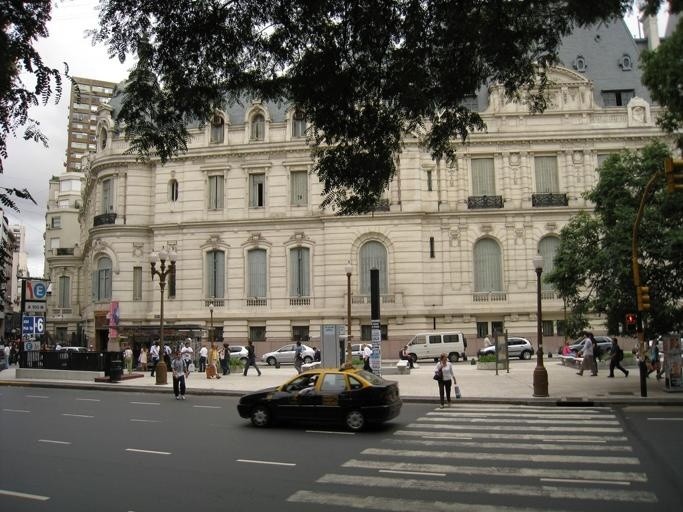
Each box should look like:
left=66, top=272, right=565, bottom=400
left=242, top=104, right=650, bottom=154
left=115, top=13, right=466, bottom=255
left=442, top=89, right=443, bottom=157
left=175, top=396, right=185, bottom=400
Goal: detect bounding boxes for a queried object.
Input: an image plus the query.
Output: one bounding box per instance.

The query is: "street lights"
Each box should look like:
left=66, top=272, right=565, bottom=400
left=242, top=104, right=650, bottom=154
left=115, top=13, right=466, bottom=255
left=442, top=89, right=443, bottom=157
left=148, top=246, right=177, bottom=387
left=207, top=304, right=215, bottom=348
left=532, top=253, right=549, bottom=397
left=343, top=259, right=352, bottom=363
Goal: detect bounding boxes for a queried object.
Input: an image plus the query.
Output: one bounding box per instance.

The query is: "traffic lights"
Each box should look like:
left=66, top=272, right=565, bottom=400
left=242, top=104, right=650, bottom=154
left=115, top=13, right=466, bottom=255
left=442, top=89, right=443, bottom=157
left=663, top=157, right=682, bottom=193
left=636, top=285, right=650, bottom=313
left=626, top=313, right=636, bottom=324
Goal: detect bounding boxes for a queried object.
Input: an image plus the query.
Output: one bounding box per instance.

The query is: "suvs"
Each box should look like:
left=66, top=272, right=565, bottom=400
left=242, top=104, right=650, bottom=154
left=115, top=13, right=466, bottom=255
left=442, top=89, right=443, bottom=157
left=55, top=346, right=87, bottom=360
left=557, top=335, right=613, bottom=357
left=217, top=346, right=249, bottom=366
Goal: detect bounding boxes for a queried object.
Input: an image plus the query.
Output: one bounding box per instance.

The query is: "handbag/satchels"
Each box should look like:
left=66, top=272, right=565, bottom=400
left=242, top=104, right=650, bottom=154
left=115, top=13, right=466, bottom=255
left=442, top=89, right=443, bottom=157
left=432, top=361, right=444, bottom=380
left=454, top=384, right=461, bottom=399
left=614, top=346, right=623, bottom=360
left=206, top=364, right=217, bottom=375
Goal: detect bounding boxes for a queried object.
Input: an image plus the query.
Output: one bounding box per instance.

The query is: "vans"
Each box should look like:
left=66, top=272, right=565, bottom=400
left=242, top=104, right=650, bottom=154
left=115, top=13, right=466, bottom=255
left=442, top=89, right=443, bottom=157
left=399, top=331, right=466, bottom=363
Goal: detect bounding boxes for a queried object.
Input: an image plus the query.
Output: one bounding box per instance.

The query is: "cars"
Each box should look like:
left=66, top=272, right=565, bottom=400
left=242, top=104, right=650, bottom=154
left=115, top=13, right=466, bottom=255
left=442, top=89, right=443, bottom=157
left=236, top=363, right=401, bottom=434
left=259, top=343, right=315, bottom=366
left=477, top=337, right=533, bottom=360
left=345, top=343, right=373, bottom=360
left=629, top=334, right=682, bottom=354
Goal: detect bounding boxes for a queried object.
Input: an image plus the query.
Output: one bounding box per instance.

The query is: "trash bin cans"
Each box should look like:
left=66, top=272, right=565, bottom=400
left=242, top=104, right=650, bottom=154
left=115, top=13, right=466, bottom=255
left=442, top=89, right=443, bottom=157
left=111, top=360, right=122, bottom=380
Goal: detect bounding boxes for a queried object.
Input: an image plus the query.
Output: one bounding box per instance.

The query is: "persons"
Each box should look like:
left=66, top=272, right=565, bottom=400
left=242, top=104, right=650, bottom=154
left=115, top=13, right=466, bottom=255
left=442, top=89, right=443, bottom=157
left=289, top=375, right=318, bottom=402
left=122, top=340, right=261, bottom=400
left=292, top=341, right=304, bottom=375
left=482, top=334, right=491, bottom=348
left=360, top=343, right=371, bottom=373
left=562, top=332, right=668, bottom=380
left=312, top=345, right=321, bottom=362
left=433, top=353, right=457, bottom=409
left=1, top=340, right=49, bottom=369
left=398, top=345, right=414, bottom=369
